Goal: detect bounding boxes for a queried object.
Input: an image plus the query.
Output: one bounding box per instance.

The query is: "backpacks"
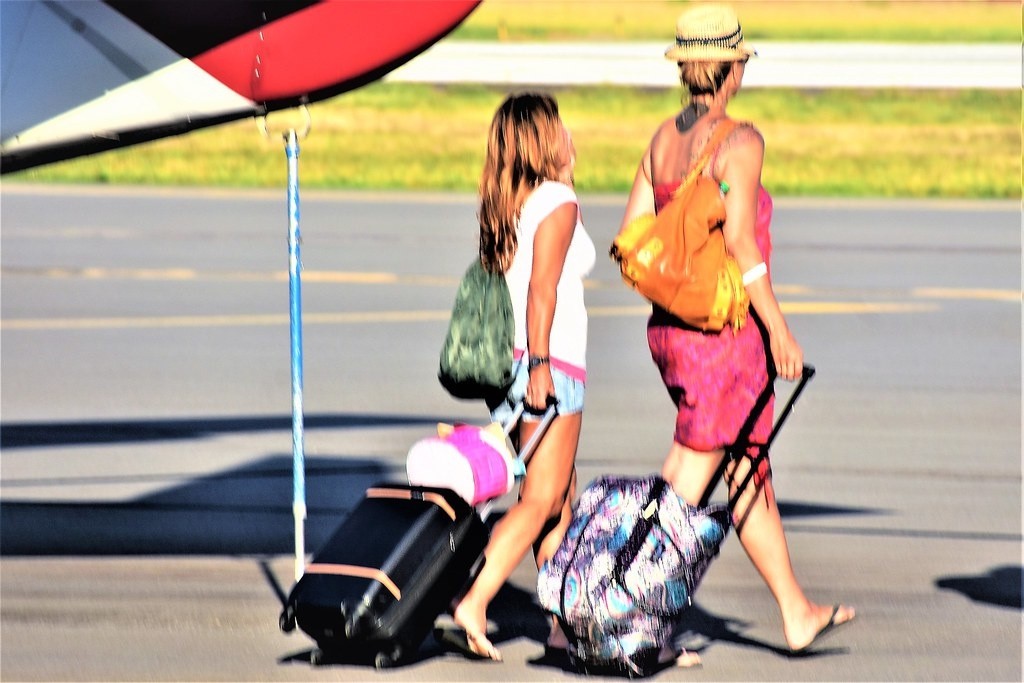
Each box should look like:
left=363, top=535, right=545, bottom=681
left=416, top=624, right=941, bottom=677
left=439, top=256, right=515, bottom=398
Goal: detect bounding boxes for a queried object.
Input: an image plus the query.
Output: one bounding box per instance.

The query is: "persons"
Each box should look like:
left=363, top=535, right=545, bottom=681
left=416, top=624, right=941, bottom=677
left=615, top=5, right=856, bottom=669
left=443, top=89, right=598, bottom=665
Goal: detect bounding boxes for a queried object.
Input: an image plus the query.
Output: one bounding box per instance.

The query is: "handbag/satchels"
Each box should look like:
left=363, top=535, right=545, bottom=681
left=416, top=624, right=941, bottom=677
left=609, top=120, right=750, bottom=331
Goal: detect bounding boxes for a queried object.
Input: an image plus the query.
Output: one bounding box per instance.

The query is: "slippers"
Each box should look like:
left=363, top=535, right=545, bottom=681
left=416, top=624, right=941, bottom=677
left=434, top=625, right=503, bottom=661
left=789, top=603, right=855, bottom=655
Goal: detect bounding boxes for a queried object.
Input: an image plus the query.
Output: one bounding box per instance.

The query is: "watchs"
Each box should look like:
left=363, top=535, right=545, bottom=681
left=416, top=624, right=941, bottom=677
left=527, top=357, right=551, bottom=371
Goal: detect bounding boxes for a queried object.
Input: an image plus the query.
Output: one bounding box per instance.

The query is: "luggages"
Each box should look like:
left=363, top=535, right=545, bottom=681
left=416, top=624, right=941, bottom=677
left=538, top=363, right=817, bottom=679
left=280, top=395, right=557, bottom=665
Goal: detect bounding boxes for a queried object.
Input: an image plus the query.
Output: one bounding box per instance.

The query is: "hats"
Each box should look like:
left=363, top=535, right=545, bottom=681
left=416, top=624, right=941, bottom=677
left=665, top=6, right=757, bottom=62
